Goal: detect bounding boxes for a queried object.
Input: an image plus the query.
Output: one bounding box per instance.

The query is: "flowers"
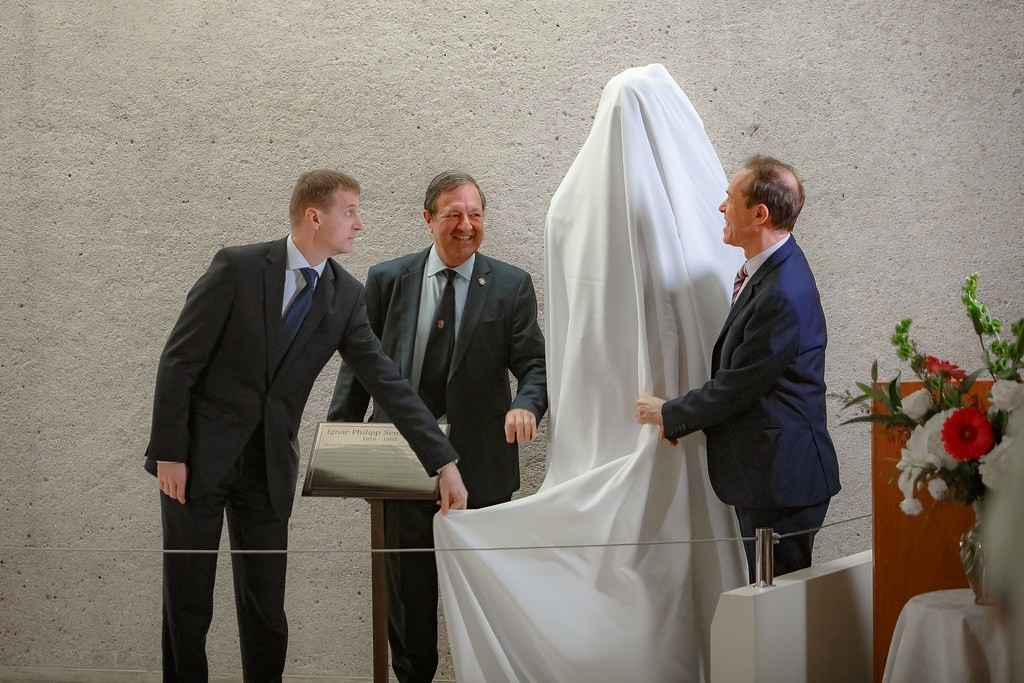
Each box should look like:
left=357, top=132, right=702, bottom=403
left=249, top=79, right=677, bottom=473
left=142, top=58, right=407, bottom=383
left=827, top=271, right=1024, bottom=515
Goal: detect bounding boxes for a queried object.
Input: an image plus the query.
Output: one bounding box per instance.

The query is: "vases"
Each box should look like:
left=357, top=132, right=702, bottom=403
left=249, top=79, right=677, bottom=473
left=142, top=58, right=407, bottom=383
left=958, top=496, right=990, bottom=605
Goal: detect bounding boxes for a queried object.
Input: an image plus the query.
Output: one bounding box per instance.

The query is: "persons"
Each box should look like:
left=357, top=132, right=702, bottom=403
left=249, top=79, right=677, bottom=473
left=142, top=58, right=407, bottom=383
left=144, top=168, right=467, bottom=683
left=634, top=155, right=842, bottom=585
left=327, top=170, right=548, bottom=683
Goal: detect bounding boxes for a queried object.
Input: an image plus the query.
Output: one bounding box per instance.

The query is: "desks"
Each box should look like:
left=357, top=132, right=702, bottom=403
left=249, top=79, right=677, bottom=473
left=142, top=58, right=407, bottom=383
left=882, top=589, right=1024, bottom=683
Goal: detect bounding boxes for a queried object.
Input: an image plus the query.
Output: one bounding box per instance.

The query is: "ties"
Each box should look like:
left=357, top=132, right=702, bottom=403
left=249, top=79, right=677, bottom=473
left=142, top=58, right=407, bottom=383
left=417, top=268, right=457, bottom=419
left=730, top=264, right=748, bottom=308
left=277, top=268, right=319, bottom=372
